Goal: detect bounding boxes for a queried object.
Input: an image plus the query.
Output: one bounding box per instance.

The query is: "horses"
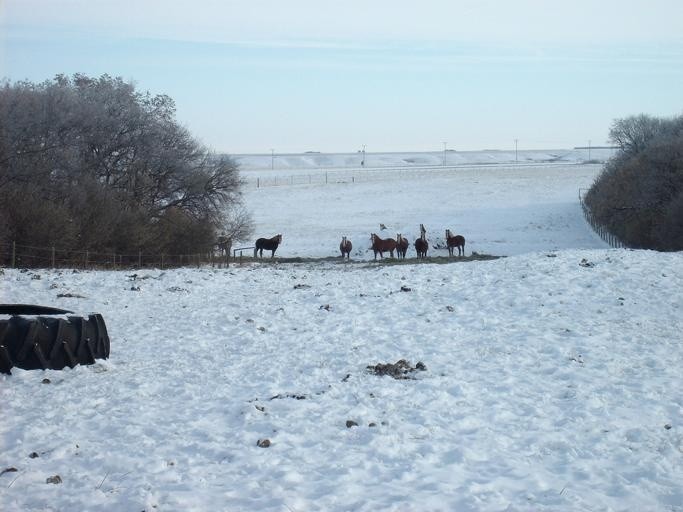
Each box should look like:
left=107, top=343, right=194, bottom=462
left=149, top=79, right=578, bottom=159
left=339, top=236, right=353, bottom=261
left=415, top=226, right=429, bottom=258
left=445, top=229, right=466, bottom=257
left=255, top=234, right=282, bottom=258
left=396, top=233, right=409, bottom=260
left=370, top=233, right=396, bottom=260
